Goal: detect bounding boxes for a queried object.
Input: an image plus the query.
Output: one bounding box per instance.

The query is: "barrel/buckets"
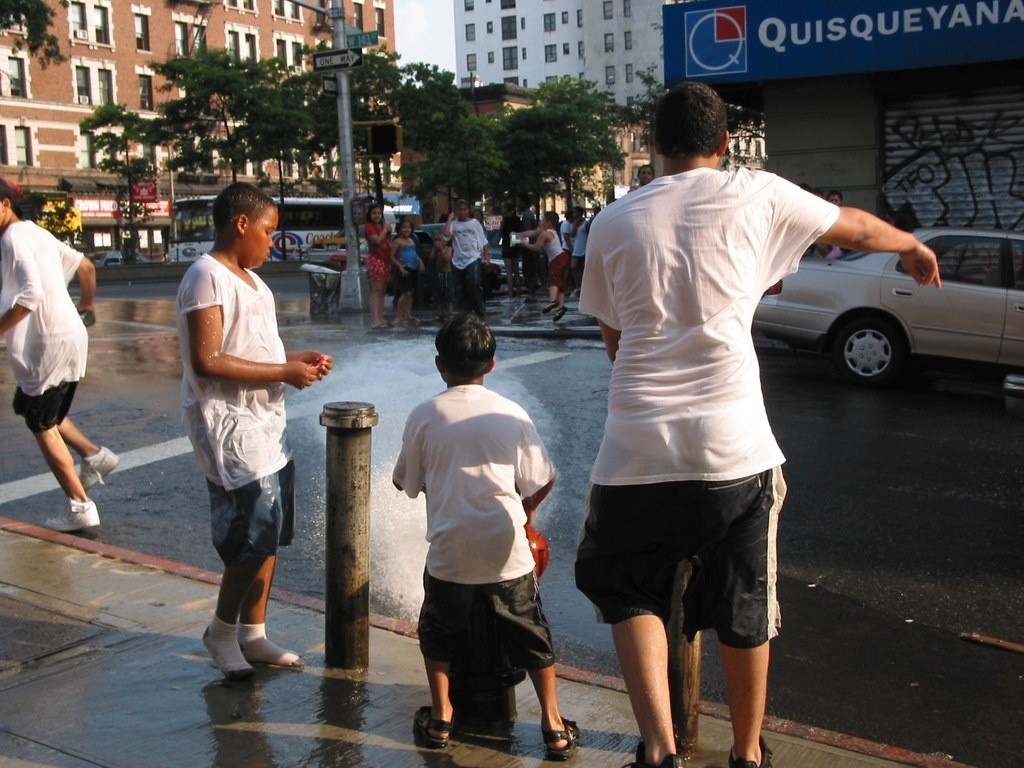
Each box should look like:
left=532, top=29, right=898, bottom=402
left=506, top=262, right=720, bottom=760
left=510, top=234, right=529, bottom=247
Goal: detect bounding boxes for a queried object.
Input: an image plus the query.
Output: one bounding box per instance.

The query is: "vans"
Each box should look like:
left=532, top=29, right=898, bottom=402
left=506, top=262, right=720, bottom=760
left=100, top=251, right=143, bottom=266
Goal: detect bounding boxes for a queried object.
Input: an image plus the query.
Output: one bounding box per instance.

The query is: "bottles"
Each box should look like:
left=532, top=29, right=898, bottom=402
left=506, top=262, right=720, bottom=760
left=79, top=309, right=95, bottom=326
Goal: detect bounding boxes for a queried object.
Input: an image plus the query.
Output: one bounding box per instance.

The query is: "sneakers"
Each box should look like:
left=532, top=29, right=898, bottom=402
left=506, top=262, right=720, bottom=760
left=543, top=300, right=560, bottom=313
left=46, top=496, right=100, bottom=530
left=552, top=306, right=568, bottom=321
left=80, top=446, right=120, bottom=490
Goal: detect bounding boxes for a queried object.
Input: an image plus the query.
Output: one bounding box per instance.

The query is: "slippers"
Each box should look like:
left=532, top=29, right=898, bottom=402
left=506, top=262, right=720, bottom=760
left=372, top=321, right=391, bottom=329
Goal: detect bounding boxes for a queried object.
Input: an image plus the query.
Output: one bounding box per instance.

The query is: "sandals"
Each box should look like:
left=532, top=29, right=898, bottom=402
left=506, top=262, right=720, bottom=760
left=542, top=716, right=579, bottom=761
left=415, top=706, right=452, bottom=747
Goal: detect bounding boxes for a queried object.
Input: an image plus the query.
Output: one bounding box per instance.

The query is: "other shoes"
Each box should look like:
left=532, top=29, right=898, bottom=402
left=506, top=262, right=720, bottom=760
left=621, top=742, right=683, bottom=768
left=728, top=736, right=773, bottom=768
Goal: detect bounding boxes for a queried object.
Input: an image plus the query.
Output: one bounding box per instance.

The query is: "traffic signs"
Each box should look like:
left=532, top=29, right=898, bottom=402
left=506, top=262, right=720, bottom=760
left=312, top=46, right=364, bottom=72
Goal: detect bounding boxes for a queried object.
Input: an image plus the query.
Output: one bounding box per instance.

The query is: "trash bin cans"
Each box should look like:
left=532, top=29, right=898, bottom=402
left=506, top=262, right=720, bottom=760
left=309, top=270, right=341, bottom=322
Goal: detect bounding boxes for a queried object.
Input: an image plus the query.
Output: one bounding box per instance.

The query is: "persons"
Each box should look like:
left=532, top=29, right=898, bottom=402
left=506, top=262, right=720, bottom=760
left=176, top=181, right=334, bottom=681
left=393, top=313, right=580, bottom=759
left=388, top=219, right=424, bottom=323
left=365, top=206, right=392, bottom=329
left=628, top=165, right=654, bottom=192
left=573, top=81, right=942, bottom=768
left=796, top=182, right=850, bottom=258
left=0, top=179, right=120, bottom=532
left=499, top=195, right=602, bottom=299
left=428, top=198, right=499, bottom=322
left=514, top=211, right=569, bottom=321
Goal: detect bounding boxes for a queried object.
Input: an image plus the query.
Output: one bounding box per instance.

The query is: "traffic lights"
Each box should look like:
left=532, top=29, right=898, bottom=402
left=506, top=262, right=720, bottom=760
left=371, top=125, right=397, bottom=155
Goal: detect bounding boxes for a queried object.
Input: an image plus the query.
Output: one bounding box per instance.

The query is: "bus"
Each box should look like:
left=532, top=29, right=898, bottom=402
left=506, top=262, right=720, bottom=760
left=167, top=194, right=420, bottom=262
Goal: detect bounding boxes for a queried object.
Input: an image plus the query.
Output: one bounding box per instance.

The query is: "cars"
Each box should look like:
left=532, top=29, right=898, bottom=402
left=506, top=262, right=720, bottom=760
left=359, top=222, right=536, bottom=296
left=750, top=228, right=1024, bottom=393
